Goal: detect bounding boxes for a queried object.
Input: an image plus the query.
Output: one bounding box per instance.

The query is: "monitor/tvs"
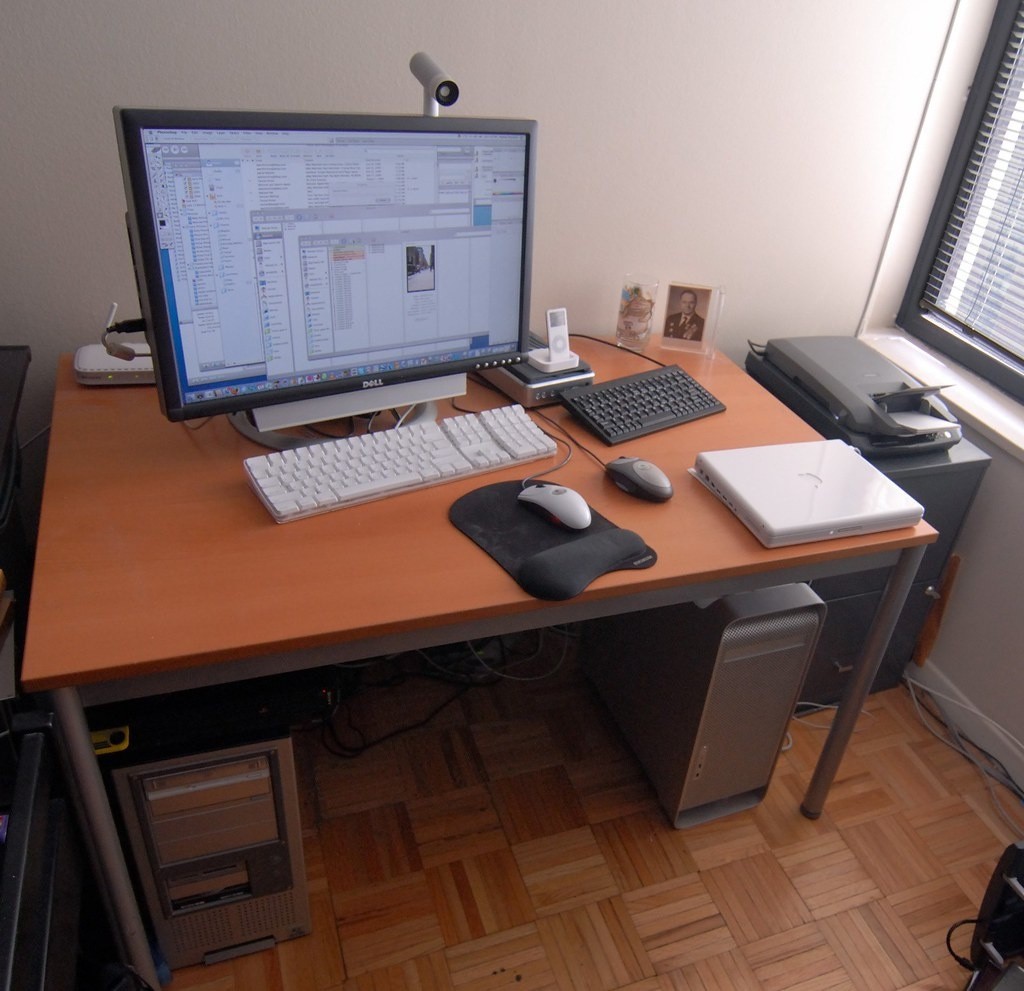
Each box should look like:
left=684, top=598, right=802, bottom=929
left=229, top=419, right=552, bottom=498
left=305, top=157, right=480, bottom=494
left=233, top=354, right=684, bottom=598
left=112, top=105, right=539, bottom=452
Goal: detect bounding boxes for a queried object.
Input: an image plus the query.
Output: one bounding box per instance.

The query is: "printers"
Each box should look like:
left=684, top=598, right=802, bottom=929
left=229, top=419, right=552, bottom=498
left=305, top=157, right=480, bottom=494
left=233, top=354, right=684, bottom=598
left=745, top=335, right=963, bottom=457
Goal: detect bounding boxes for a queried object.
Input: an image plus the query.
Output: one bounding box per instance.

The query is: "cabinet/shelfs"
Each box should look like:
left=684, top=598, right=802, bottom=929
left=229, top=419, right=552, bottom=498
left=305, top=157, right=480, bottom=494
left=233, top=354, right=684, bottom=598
left=741, top=350, right=993, bottom=717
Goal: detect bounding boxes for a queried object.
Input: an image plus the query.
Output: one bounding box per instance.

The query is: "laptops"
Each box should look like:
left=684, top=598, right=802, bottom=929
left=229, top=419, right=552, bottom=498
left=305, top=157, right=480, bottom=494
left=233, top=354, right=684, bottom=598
left=695, top=438, right=924, bottom=550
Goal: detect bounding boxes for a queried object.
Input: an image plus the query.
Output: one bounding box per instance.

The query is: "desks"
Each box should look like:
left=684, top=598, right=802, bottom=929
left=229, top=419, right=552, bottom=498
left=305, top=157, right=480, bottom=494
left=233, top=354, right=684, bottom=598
left=19, top=333, right=941, bottom=991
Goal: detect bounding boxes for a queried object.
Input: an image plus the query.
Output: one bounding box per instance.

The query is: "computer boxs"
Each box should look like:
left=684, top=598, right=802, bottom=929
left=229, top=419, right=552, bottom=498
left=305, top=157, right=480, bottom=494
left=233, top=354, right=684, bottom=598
left=111, top=707, right=313, bottom=969
left=576, top=581, right=828, bottom=829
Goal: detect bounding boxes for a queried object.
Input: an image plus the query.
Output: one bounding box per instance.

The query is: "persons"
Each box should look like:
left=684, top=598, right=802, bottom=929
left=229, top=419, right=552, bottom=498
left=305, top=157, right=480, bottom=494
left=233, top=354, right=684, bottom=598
left=664, top=291, right=704, bottom=341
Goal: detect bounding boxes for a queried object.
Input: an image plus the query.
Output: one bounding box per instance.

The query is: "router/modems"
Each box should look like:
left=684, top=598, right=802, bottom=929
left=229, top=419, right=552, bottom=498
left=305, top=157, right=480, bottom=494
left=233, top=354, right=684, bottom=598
left=73, top=342, right=157, bottom=385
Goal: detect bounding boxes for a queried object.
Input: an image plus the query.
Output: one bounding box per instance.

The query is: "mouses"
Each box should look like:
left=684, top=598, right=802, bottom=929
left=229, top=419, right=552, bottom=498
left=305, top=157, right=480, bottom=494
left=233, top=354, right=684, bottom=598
left=605, top=456, right=674, bottom=502
left=517, top=483, right=592, bottom=531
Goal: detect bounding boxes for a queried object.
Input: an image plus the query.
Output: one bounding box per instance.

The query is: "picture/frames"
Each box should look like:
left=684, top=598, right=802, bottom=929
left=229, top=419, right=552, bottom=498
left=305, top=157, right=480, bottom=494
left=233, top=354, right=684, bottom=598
left=653, top=279, right=725, bottom=358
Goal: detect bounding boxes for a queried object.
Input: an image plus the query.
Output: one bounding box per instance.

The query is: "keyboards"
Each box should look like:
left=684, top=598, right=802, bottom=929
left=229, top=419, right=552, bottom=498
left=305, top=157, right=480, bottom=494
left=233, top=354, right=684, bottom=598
left=243, top=405, right=558, bottom=524
left=560, top=363, right=726, bottom=447
